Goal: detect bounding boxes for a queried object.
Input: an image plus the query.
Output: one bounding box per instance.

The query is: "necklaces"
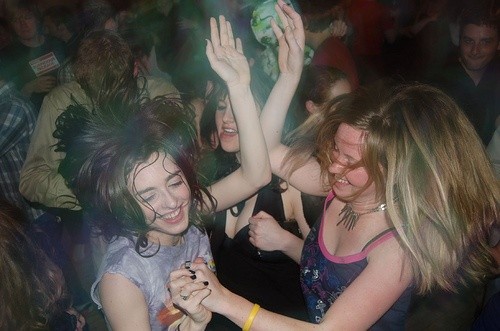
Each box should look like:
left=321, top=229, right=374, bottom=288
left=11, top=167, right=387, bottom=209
left=336, top=195, right=399, bottom=232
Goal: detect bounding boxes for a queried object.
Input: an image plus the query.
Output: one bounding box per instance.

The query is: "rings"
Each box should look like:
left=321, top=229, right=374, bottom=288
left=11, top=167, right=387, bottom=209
left=179, top=289, right=190, bottom=300
left=184, top=261, right=192, bottom=269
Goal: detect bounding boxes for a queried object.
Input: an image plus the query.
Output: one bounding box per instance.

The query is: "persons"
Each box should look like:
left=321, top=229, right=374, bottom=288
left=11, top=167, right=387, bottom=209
left=57, top=17, right=272, bottom=331
left=192, top=71, right=312, bottom=331
left=1, top=203, right=87, bottom=330
left=0, top=78, right=94, bottom=314
left=1, top=1, right=499, bottom=183
left=166, top=1, right=499, bottom=330
left=18, top=30, right=183, bottom=281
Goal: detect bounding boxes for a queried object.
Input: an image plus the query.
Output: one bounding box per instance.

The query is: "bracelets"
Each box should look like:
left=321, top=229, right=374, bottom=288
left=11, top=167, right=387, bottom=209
left=241, top=303, right=261, bottom=331
